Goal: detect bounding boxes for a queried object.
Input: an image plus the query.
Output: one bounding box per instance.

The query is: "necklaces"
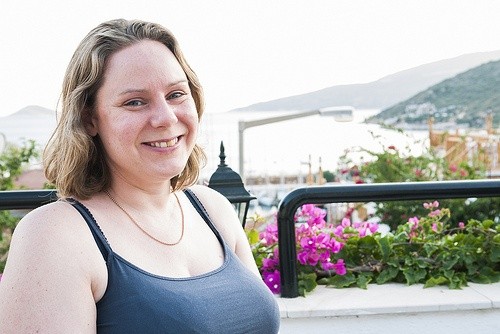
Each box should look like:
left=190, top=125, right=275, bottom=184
left=103, top=188, right=184, bottom=246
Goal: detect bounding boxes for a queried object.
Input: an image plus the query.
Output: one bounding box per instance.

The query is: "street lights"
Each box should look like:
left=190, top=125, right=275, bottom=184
left=238, top=106, right=354, bottom=183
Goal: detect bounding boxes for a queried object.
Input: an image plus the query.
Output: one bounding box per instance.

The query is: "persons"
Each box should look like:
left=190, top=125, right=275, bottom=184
left=0, top=19, right=279, bottom=334
